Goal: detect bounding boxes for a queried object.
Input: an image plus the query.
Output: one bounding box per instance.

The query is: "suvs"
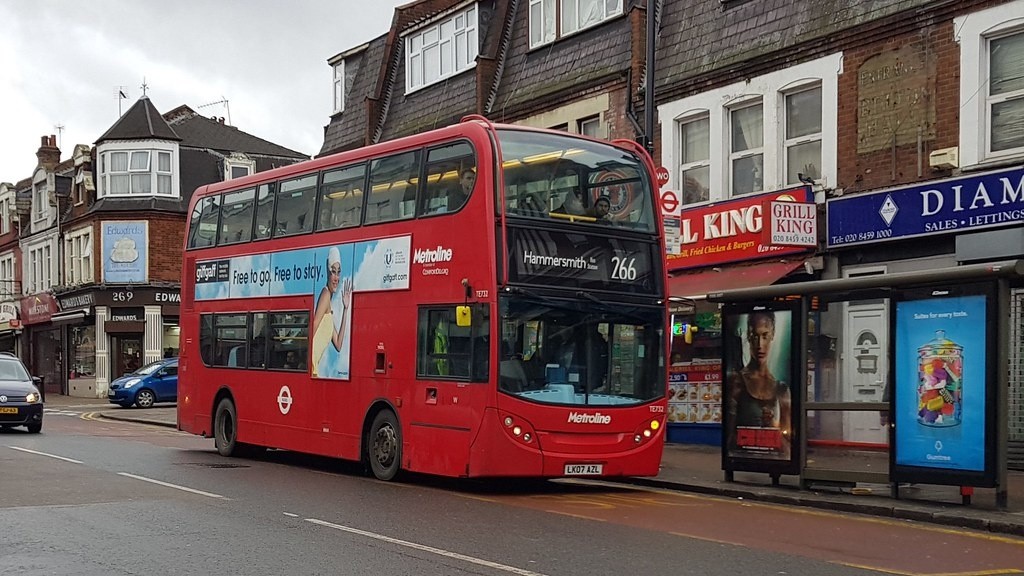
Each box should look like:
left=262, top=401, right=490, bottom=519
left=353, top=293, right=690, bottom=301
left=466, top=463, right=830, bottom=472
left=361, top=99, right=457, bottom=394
left=0, top=353, right=43, bottom=434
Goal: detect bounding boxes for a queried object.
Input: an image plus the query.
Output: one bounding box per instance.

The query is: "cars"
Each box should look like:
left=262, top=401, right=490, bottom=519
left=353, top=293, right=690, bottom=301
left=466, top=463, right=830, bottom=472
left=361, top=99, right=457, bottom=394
left=108, top=358, right=178, bottom=409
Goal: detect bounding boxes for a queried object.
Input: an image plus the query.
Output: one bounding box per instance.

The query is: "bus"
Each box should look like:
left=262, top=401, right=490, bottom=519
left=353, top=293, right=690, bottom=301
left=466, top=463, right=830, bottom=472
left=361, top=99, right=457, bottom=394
left=179, top=115, right=673, bottom=484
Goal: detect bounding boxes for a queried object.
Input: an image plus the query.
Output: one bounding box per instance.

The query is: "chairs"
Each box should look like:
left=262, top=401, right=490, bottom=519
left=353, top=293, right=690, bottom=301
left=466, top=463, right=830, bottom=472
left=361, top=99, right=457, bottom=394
left=192, top=212, right=322, bottom=246
left=227, top=345, right=259, bottom=365
left=273, top=348, right=300, bottom=368
